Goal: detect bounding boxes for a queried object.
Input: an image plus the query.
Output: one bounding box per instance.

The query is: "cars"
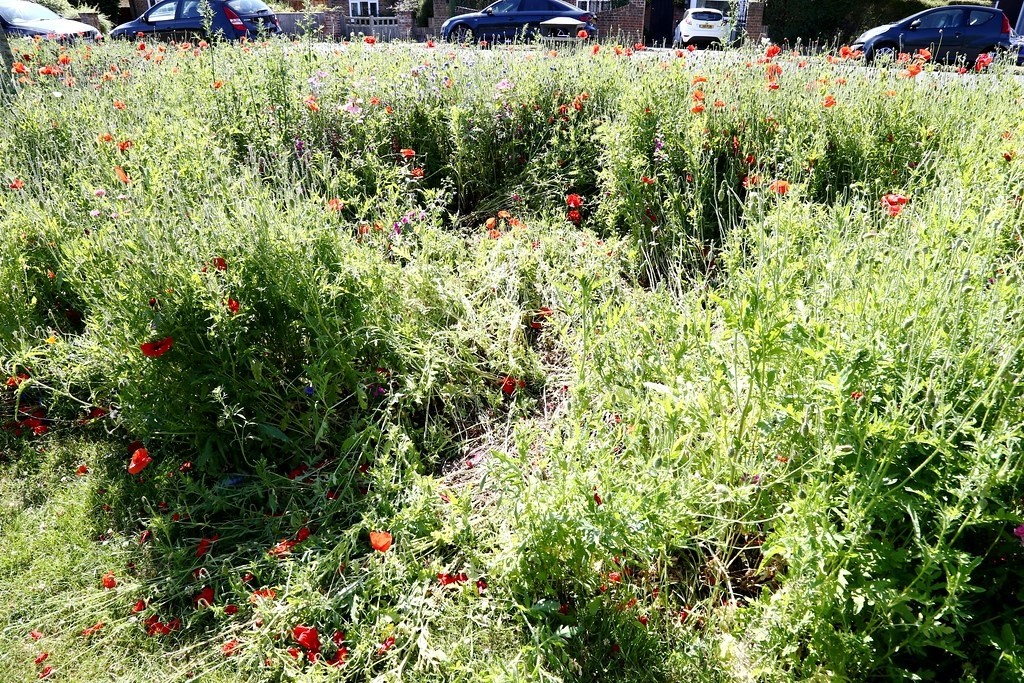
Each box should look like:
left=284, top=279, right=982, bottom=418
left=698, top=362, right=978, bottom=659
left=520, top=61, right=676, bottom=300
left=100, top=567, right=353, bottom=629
left=849, top=4, right=1011, bottom=74
left=674, top=7, right=733, bottom=51
left=438, top=0, right=599, bottom=48
left=108, top=0, right=282, bottom=47
left=1009, top=27, right=1024, bottom=66
left=1, top=0, right=105, bottom=49
